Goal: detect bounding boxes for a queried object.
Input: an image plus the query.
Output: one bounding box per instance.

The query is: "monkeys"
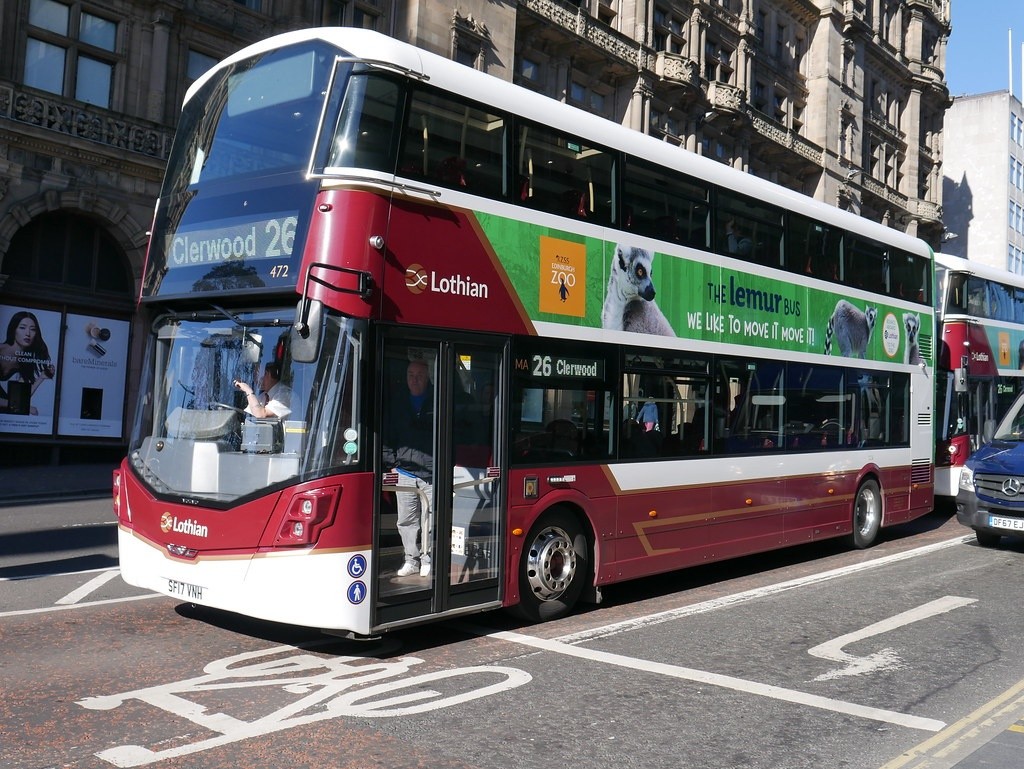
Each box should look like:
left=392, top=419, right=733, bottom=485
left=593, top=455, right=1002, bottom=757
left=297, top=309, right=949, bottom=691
left=601, top=242, right=677, bottom=340
left=903, top=313, right=926, bottom=366
left=823, top=299, right=877, bottom=359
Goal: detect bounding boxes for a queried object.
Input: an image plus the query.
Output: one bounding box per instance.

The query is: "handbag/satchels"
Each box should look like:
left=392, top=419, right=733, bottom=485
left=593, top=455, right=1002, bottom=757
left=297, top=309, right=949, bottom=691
left=654, top=424, right=661, bottom=432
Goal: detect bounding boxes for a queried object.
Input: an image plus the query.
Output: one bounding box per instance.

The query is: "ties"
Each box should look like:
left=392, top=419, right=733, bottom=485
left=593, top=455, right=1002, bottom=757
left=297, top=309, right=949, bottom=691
left=264, top=392, right=270, bottom=408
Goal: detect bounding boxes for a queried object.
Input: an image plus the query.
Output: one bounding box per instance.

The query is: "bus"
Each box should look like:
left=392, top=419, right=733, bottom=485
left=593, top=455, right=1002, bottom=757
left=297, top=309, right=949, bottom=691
left=932, top=248, right=1024, bottom=496
left=113, top=26, right=938, bottom=642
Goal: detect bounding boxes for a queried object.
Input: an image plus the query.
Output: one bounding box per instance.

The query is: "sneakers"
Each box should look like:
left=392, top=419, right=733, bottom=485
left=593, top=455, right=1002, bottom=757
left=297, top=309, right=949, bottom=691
left=397, top=563, right=420, bottom=576
left=420, top=564, right=431, bottom=576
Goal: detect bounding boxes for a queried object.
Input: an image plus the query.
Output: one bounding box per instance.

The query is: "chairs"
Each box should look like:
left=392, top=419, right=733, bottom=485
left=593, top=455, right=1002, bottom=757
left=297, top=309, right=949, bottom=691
left=400, top=147, right=880, bottom=294
left=460, top=413, right=861, bottom=467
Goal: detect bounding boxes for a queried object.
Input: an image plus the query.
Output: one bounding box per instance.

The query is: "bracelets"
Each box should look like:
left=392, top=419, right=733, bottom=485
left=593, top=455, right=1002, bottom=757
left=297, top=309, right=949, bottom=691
left=245, top=392, right=255, bottom=397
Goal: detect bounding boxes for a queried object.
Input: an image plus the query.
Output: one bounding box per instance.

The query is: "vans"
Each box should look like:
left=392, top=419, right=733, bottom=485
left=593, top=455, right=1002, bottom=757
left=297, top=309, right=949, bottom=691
left=954, top=384, right=1024, bottom=548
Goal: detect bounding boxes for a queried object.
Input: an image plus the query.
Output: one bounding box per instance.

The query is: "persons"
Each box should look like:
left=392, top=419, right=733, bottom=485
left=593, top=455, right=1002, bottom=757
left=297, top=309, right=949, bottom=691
left=268, top=450, right=269, bottom=453
left=727, top=216, right=755, bottom=259
left=0, top=311, right=55, bottom=416
left=235, top=362, right=302, bottom=437
left=386, top=360, right=579, bottom=589
left=636, top=396, right=659, bottom=432
